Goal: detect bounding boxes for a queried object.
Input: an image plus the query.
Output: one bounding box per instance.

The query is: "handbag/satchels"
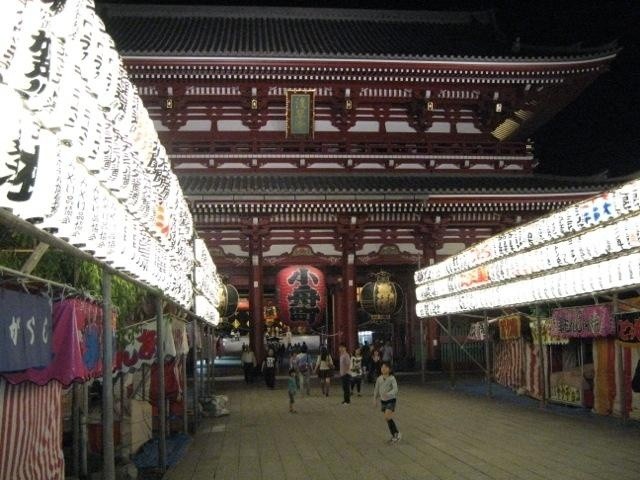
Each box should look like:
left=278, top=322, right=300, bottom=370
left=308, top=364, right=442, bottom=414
left=298, top=361, right=312, bottom=377
left=328, top=364, right=336, bottom=370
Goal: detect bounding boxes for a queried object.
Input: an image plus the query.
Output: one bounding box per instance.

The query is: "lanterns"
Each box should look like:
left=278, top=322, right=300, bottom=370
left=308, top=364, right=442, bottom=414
left=359, top=270, right=404, bottom=327
left=263, top=302, right=279, bottom=327
left=412, top=177, right=640, bottom=320
left=275, top=264, right=327, bottom=335
left=0, top=0, right=241, bottom=330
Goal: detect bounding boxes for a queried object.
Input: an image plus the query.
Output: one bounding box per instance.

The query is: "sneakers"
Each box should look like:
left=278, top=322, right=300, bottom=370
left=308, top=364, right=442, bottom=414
left=341, top=400, right=351, bottom=406
left=390, top=431, right=400, bottom=442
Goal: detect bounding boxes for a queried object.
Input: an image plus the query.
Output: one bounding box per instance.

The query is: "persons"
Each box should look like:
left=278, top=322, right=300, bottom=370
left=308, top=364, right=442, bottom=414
left=240, top=339, right=394, bottom=413
left=371, top=360, right=402, bottom=444
